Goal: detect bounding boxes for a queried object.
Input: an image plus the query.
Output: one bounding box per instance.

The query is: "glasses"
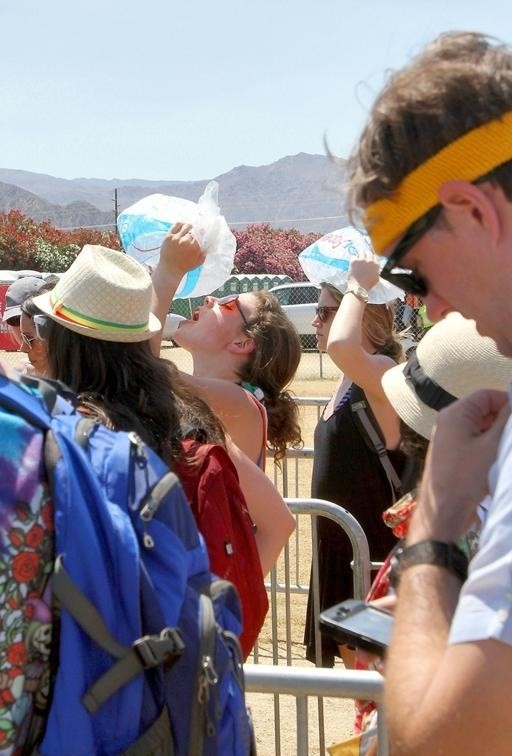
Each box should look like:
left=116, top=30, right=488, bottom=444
left=315, top=307, right=339, bottom=321
left=21, top=333, right=38, bottom=350
left=378, top=177, right=490, bottom=299
left=33, top=314, right=54, bottom=342
left=218, top=294, right=249, bottom=329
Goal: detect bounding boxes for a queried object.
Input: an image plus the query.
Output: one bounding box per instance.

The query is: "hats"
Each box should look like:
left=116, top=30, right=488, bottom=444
left=380, top=312, right=511, bottom=441
left=3, top=277, right=47, bottom=322
left=32, top=245, right=162, bottom=339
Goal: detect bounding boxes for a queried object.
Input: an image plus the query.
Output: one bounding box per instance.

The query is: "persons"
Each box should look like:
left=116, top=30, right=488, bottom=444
left=335, top=309, right=512, bottom=755
left=148, top=222, right=306, bottom=475
left=323, top=28, right=512, bottom=754
left=33, top=243, right=227, bottom=468
left=16, top=281, right=56, bottom=374
left=302, top=249, right=411, bottom=667
left=0, top=363, right=63, bottom=753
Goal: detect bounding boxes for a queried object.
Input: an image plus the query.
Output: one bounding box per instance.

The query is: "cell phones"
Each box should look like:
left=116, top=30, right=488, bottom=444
left=314, top=594, right=392, bottom=654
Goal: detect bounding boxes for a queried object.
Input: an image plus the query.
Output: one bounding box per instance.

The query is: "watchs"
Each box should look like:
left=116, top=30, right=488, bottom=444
left=341, top=285, right=370, bottom=306
